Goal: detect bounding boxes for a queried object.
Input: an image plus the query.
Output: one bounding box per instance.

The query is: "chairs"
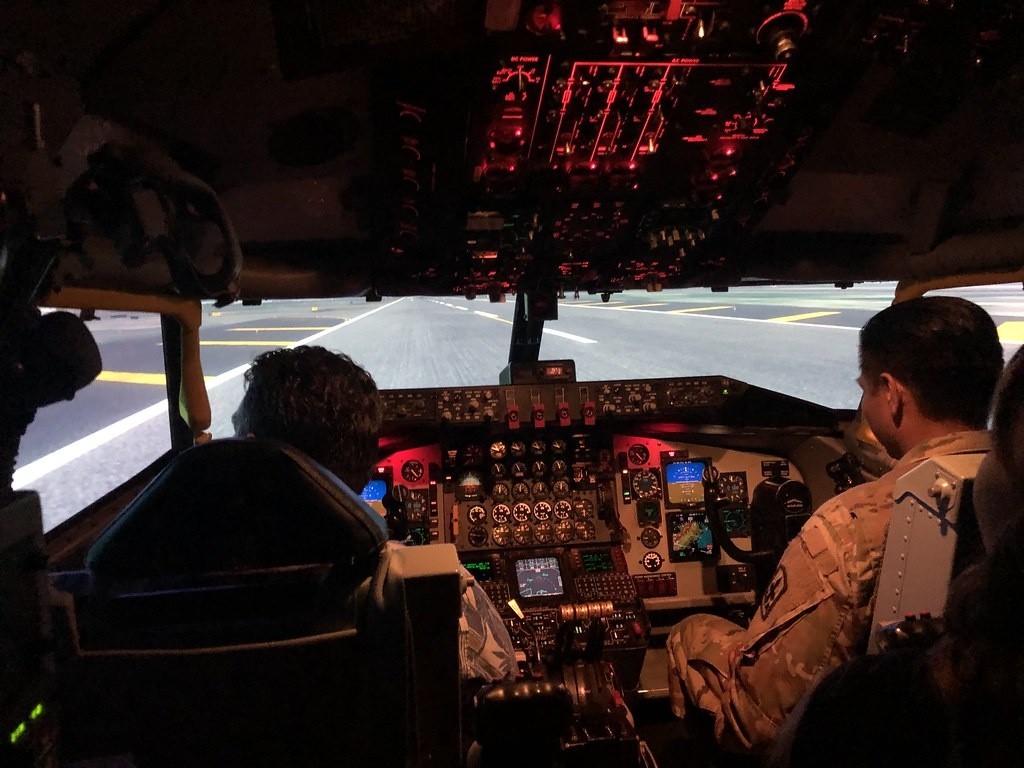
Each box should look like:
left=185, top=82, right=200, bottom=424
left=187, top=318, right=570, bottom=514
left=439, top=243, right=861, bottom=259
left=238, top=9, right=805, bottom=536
left=767, top=347, right=1024, bottom=767
left=48, top=434, right=463, bottom=767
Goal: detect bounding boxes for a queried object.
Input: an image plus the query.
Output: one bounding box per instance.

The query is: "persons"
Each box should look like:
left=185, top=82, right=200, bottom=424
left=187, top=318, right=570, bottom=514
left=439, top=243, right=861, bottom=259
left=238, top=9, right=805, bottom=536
left=765, top=345, right=1024, bottom=768
left=234, top=347, right=520, bottom=684
left=664, top=296, right=1005, bottom=768
